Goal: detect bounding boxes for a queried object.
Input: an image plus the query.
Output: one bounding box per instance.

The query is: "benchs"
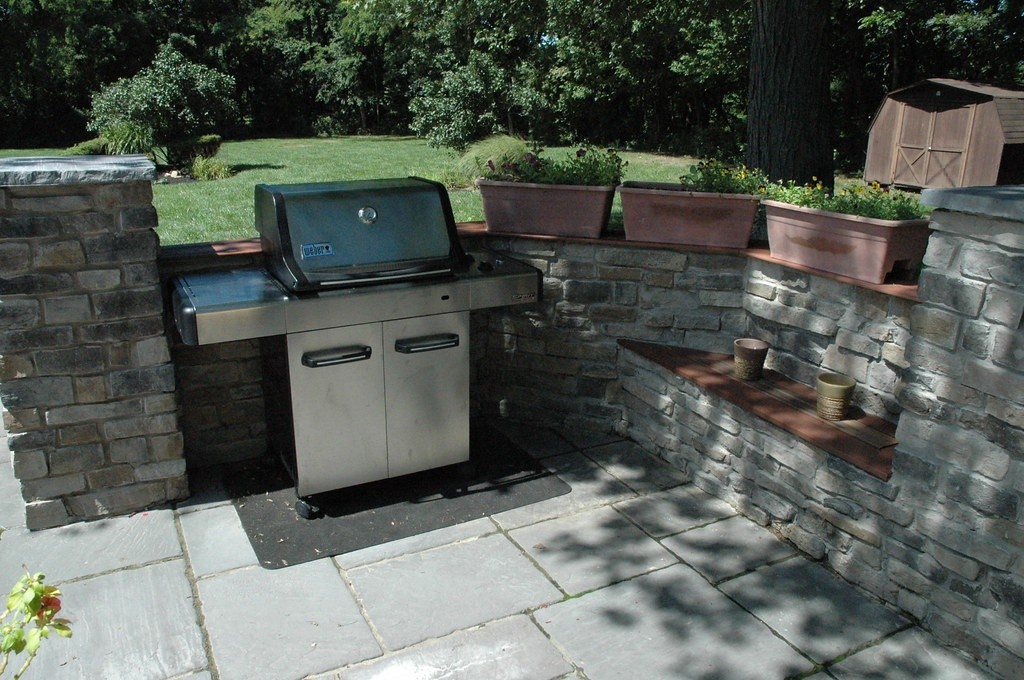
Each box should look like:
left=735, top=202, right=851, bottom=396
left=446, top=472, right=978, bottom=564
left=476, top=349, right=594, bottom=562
left=615, top=337, right=902, bottom=480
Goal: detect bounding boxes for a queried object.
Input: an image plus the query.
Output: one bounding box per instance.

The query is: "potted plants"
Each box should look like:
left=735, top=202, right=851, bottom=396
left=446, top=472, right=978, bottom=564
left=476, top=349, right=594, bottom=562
left=616, top=176, right=760, bottom=248
left=760, top=198, right=933, bottom=292
left=473, top=176, right=614, bottom=240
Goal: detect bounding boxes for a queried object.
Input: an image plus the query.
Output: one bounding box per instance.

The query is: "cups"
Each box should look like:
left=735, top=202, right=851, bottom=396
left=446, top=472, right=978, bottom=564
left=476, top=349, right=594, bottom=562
left=816, top=373, right=857, bottom=422
left=734, top=338, right=769, bottom=382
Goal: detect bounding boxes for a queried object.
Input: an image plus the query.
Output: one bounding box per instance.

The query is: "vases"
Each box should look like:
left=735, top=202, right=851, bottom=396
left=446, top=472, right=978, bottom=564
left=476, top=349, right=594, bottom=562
left=814, top=368, right=857, bottom=424
left=732, top=336, right=770, bottom=381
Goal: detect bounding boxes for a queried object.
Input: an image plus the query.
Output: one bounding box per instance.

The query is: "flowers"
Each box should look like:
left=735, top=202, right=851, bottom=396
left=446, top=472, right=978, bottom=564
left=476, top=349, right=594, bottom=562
left=694, top=158, right=765, bottom=196
left=489, top=148, right=630, bottom=188
left=766, top=173, right=935, bottom=225
